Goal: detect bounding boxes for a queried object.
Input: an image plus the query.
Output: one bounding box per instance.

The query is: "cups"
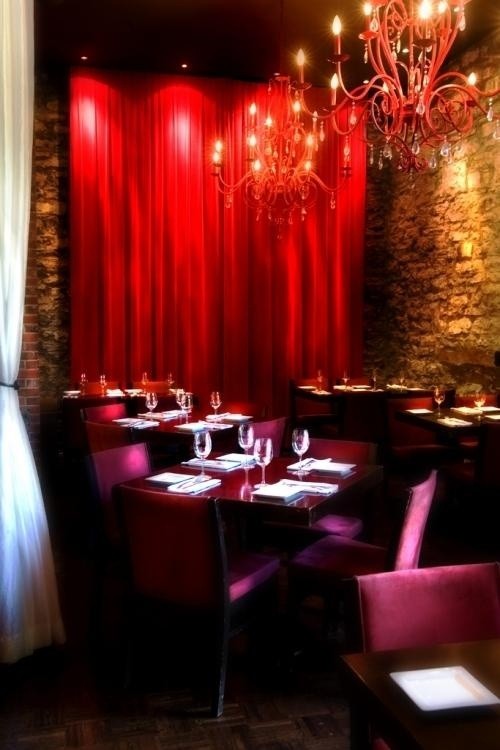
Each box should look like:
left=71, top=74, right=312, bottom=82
left=77, top=372, right=175, bottom=395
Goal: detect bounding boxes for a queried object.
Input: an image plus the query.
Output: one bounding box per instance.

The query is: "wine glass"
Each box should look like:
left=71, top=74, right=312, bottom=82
left=433, top=386, right=446, bottom=416
left=209, top=391, right=222, bottom=424
left=176, top=390, right=194, bottom=424
left=291, top=428, right=310, bottom=475
left=194, top=430, right=213, bottom=482
left=145, top=392, right=158, bottom=422
left=252, top=438, right=273, bottom=489
left=315, top=367, right=405, bottom=392
left=473, top=393, right=486, bottom=420
left=237, top=424, right=256, bottom=469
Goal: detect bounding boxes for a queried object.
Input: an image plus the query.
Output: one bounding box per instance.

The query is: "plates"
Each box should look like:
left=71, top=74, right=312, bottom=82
left=224, top=413, right=254, bottom=423
left=173, top=423, right=207, bottom=431
left=298, top=384, right=426, bottom=396
left=123, top=389, right=143, bottom=394
left=251, top=483, right=305, bottom=502
left=64, top=389, right=80, bottom=396
left=308, top=460, right=357, bottom=475
left=215, top=451, right=256, bottom=463
left=475, top=406, right=500, bottom=412
left=111, top=417, right=144, bottom=426
left=405, top=408, right=434, bottom=415
left=162, top=410, right=187, bottom=415
left=145, top=472, right=194, bottom=486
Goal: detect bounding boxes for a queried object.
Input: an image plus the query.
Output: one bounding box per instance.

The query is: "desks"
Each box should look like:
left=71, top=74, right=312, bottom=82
left=338, top=640, right=499, bottom=750
left=114, top=452, right=385, bottom=720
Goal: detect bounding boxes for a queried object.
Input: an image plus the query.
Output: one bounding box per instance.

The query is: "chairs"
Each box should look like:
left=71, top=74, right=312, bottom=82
left=354, top=562, right=499, bottom=648
left=298, top=472, right=438, bottom=647
left=116, top=482, right=289, bottom=720
left=65, top=374, right=263, bottom=459
left=244, top=416, right=287, bottom=460
left=93, top=441, right=153, bottom=529
left=287, top=374, right=500, bottom=486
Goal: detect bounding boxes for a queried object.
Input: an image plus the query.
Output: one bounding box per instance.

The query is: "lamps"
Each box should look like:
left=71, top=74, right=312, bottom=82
left=211, top=0, right=499, bottom=226
